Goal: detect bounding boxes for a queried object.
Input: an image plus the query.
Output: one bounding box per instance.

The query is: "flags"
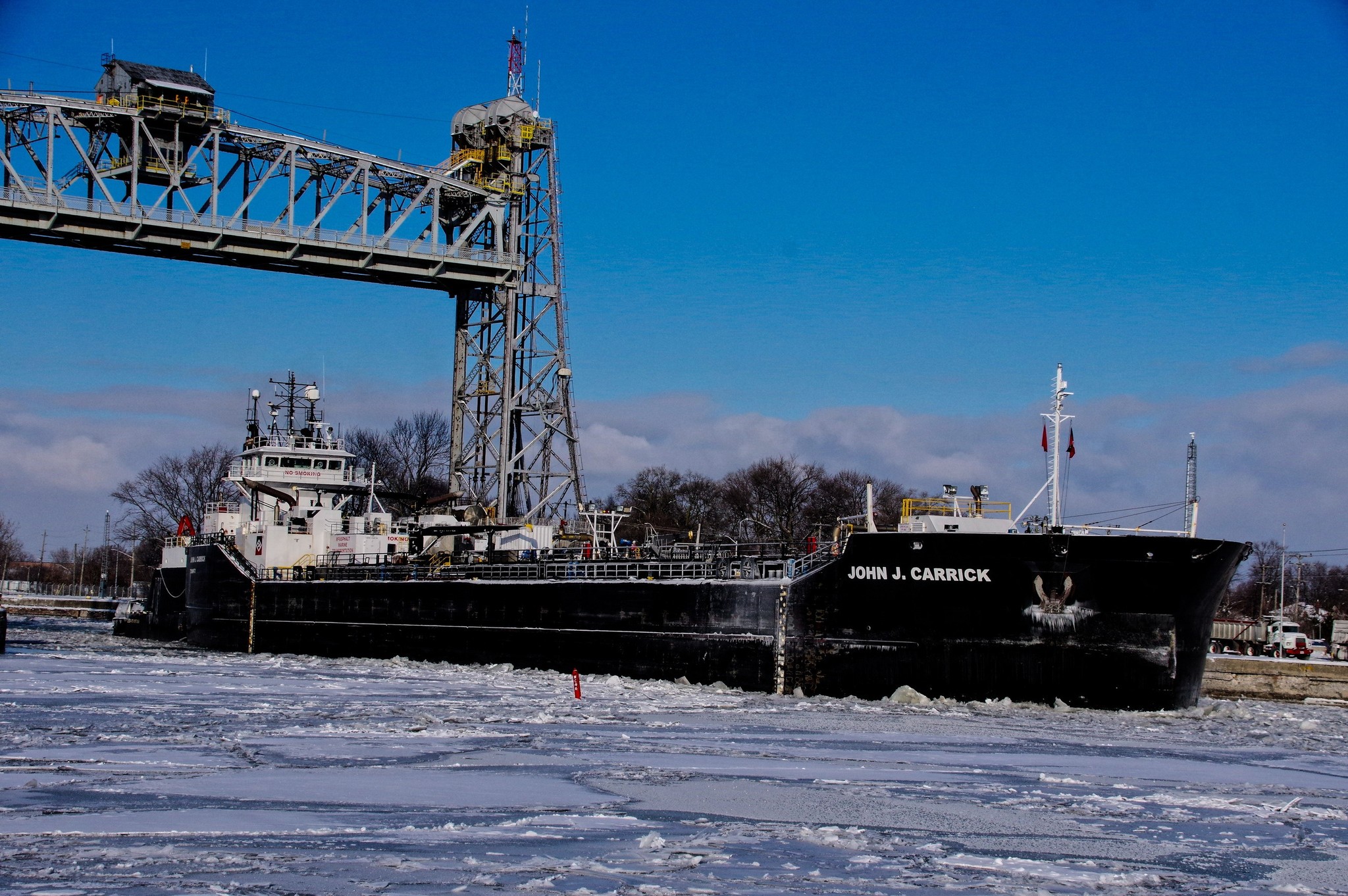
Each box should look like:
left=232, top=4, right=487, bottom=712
left=1065, top=427, right=1075, bottom=459
left=1041, top=423, right=1047, bottom=453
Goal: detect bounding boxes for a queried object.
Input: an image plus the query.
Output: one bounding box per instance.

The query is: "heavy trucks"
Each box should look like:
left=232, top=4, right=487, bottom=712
left=1208, top=615, right=1313, bottom=660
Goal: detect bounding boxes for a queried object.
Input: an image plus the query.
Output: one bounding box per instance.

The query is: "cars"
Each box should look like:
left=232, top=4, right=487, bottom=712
left=1310, top=639, right=1326, bottom=648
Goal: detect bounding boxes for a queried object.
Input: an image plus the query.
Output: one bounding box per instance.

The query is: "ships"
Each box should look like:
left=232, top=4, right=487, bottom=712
left=113, top=362, right=1253, bottom=712
